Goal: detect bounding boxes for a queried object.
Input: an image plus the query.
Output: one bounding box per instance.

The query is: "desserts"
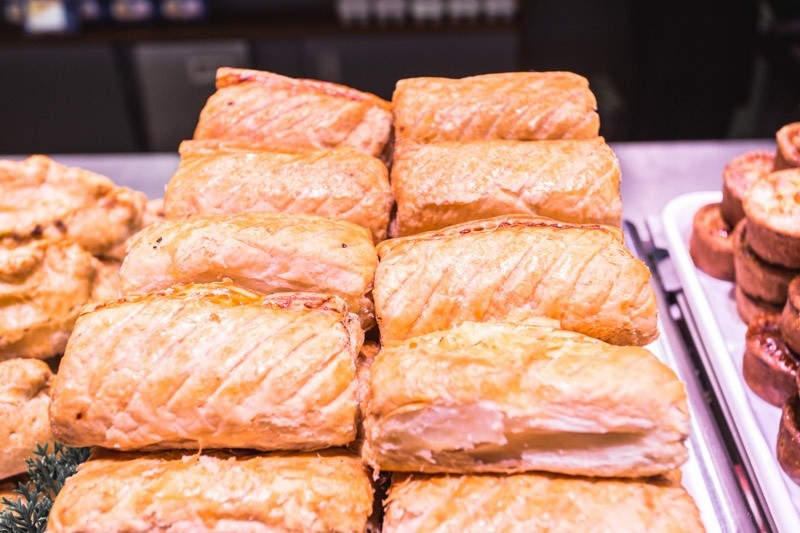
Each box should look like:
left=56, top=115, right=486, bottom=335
left=690, top=119, right=800, bottom=493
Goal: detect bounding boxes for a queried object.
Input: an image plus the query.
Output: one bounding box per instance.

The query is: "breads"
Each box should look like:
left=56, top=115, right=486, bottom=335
left=0, top=68, right=706, bottom=533
